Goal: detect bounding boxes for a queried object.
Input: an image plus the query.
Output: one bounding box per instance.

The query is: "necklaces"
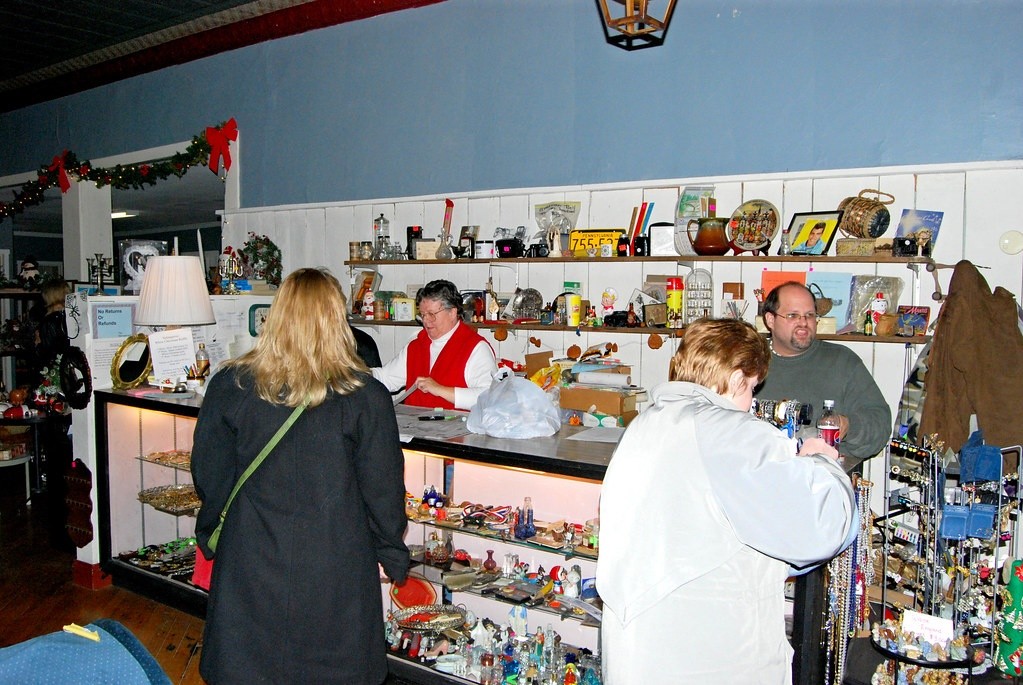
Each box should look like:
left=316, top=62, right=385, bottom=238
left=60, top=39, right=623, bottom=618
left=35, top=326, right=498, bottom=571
left=819, top=478, right=874, bottom=685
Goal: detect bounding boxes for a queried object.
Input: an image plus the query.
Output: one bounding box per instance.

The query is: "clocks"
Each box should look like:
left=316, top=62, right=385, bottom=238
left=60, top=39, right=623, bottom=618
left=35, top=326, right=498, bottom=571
left=249, top=304, right=274, bottom=337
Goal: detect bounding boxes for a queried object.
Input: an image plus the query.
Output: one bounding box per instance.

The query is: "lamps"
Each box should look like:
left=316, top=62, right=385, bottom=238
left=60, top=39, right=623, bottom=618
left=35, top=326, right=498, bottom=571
left=133, top=255, right=216, bottom=330
left=112, top=208, right=140, bottom=219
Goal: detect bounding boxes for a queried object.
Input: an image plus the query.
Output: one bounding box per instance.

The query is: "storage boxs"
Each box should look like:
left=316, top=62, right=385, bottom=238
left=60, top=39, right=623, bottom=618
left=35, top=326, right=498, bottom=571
left=558, top=388, right=636, bottom=416
left=754, top=315, right=837, bottom=335
left=347, top=270, right=382, bottom=315
left=553, top=359, right=632, bottom=375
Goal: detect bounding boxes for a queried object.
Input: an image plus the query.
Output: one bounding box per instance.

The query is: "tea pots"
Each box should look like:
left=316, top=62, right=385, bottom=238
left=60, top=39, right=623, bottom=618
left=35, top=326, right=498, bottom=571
left=435, top=228, right=454, bottom=260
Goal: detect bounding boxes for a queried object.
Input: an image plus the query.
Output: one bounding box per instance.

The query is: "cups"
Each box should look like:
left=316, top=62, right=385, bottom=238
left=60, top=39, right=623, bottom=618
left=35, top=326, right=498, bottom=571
left=564, top=294, right=582, bottom=327
left=600, top=244, right=612, bottom=257
left=187, top=375, right=205, bottom=391
left=373, top=299, right=384, bottom=320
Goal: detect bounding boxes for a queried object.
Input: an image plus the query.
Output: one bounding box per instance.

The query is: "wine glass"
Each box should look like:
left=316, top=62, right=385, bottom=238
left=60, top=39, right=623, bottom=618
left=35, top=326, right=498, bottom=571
left=586, top=248, right=597, bottom=257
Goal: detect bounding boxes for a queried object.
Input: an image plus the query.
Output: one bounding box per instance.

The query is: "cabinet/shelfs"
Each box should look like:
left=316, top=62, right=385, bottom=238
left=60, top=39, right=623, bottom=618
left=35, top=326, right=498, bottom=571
left=0, top=292, right=46, bottom=494
left=93, top=385, right=826, bottom=685
left=344, top=255, right=934, bottom=344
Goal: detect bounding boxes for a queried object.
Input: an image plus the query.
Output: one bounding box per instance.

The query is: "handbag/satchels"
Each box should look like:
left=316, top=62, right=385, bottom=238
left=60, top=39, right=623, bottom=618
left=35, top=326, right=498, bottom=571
left=465, top=365, right=562, bottom=440
left=206, top=521, right=224, bottom=552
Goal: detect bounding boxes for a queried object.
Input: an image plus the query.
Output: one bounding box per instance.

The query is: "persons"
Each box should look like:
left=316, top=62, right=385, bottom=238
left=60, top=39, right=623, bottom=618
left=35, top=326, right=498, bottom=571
left=361, top=288, right=375, bottom=320
left=906, top=230, right=931, bottom=257
left=601, top=287, right=618, bottom=323
left=190, top=266, right=409, bottom=685
left=367, top=278, right=497, bottom=605
left=31, top=277, right=72, bottom=514
left=793, top=221, right=826, bottom=255
left=595, top=317, right=855, bottom=685
left=753, top=282, right=891, bottom=475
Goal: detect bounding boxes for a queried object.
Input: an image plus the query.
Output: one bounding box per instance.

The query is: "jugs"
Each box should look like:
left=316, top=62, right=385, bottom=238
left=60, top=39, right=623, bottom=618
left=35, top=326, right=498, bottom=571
left=686, top=217, right=731, bottom=256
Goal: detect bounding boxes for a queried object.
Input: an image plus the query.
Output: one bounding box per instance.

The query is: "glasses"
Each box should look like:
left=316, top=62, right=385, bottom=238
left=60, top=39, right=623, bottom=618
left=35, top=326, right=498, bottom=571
left=416, top=306, right=451, bottom=324
left=771, top=311, right=820, bottom=323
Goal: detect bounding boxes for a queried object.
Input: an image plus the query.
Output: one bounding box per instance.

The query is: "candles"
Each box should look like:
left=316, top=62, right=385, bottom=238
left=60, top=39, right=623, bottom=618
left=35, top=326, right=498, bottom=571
left=174, top=236, right=179, bottom=256
left=197, top=229, right=206, bottom=279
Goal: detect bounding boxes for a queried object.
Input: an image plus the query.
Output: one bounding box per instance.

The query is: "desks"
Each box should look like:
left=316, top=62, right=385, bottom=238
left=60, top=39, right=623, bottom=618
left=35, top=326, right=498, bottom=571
left=0, top=454, right=32, bottom=506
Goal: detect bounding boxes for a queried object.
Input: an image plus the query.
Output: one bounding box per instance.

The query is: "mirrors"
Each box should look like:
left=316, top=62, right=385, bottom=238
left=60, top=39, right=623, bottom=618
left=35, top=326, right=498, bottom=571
left=110, top=332, right=152, bottom=391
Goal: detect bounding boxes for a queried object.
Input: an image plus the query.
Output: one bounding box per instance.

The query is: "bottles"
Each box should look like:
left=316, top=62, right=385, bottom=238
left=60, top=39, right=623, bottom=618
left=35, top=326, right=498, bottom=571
left=348, top=212, right=474, bottom=260
left=817, top=400, right=840, bottom=464
left=406, top=485, right=598, bottom=599
left=864, top=310, right=872, bottom=336
left=634, top=233, right=649, bottom=256
left=872, top=293, right=888, bottom=335
left=196, top=343, right=210, bottom=375
left=781, top=229, right=790, bottom=256
left=617, top=234, right=630, bottom=257
left=463, top=278, right=707, bottom=328
left=385, top=608, right=605, bottom=685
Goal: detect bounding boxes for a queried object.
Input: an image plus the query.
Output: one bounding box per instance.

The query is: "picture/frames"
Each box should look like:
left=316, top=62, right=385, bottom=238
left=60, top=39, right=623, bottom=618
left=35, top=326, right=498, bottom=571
left=777, top=211, right=844, bottom=255
